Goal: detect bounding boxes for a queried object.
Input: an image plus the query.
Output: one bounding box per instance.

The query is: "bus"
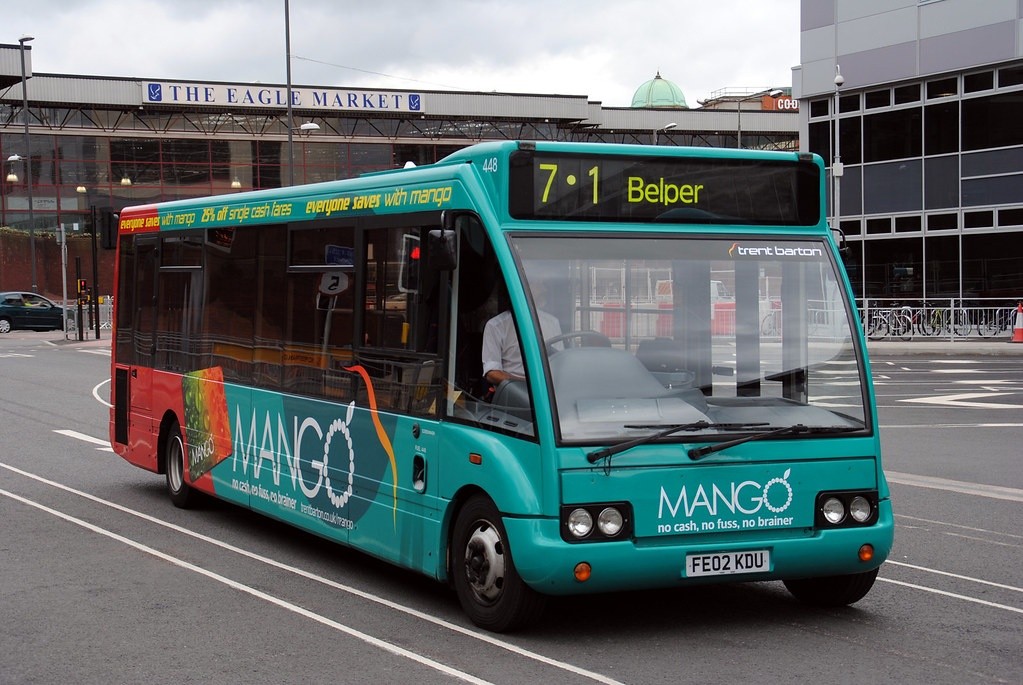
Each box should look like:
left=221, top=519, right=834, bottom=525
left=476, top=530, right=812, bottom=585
left=107, top=134, right=896, bottom=635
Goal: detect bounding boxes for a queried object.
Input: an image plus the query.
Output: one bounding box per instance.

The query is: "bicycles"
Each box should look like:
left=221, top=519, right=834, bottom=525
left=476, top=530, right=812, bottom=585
left=860, top=301, right=1018, bottom=342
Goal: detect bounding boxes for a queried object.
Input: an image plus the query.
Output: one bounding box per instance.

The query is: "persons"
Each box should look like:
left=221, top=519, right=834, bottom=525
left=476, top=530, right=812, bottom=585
left=482, top=275, right=565, bottom=390
left=23, top=296, right=42, bottom=306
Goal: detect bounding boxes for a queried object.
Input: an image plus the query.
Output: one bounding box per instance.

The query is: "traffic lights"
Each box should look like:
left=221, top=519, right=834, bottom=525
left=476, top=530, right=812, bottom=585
left=78, top=279, right=86, bottom=294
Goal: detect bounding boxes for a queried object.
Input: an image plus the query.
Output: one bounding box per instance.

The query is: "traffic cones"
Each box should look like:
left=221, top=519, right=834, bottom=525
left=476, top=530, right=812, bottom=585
left=1011, top=302, right=1023, bottom=343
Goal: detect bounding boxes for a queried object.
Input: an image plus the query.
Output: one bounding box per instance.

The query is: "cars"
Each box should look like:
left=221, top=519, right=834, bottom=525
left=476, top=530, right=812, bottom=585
left=0, top=291, right=78, bottom=333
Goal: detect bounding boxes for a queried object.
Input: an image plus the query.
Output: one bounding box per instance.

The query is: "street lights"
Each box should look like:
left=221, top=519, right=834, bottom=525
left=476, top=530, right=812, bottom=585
left=654, top=122, right=677, bottom=145
left=284, top=0, right=320, bottom=187
left=6, top=33, right=38, bottom=293
left=830, top=63, right=845, bottom=252
